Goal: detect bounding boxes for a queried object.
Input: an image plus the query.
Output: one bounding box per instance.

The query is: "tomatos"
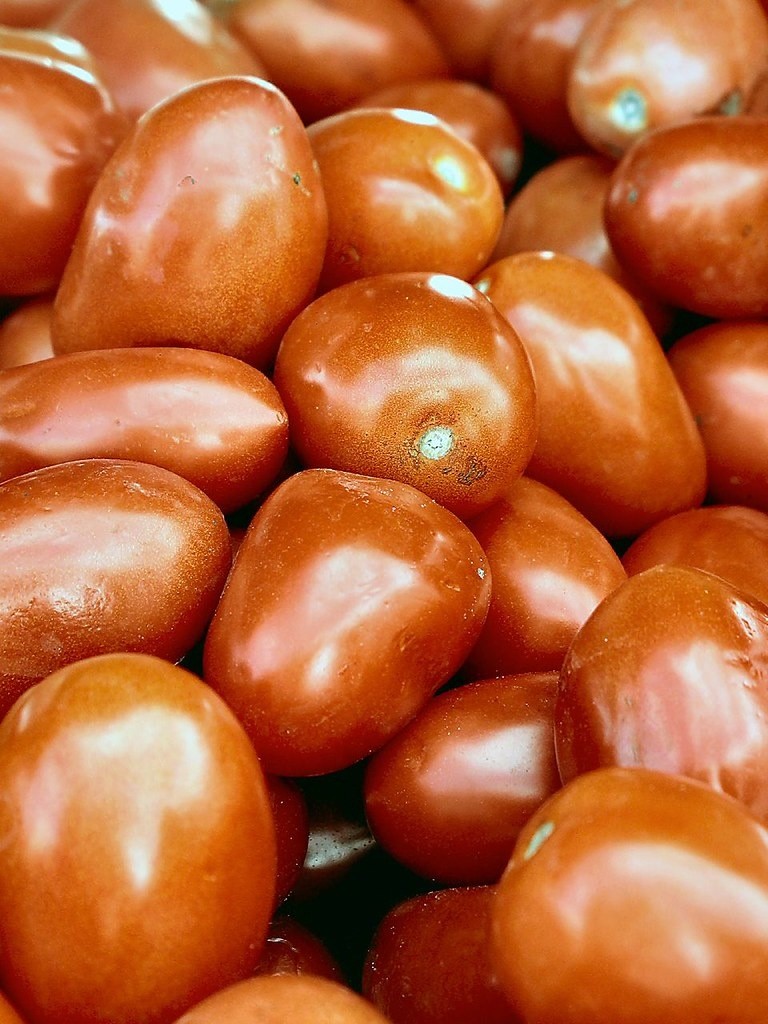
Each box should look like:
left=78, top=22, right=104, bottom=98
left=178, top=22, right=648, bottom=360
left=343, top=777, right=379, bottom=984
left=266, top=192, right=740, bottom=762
left=0, top=0, right=768, bottom=1024
